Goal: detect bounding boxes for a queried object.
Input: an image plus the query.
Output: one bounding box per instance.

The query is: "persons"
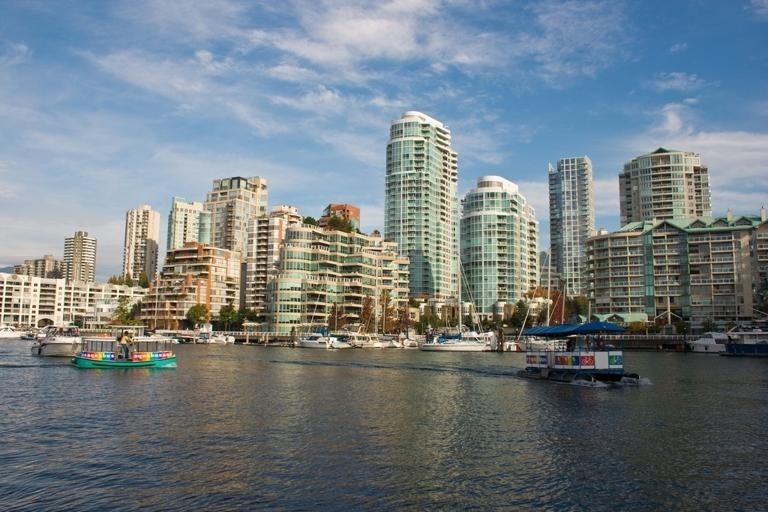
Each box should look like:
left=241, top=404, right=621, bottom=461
left=584, top=335, right=592, bottom=350
left=120, top=331, right=134, bottom=360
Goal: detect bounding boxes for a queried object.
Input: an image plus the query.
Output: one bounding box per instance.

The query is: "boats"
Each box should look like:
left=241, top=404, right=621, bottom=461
left=503, top=335, right=568, bottom=352
left=420, top=332, right=490, bottom=352
left=179, top=318, right=235, bottom=345
left=70, top=323, right=178, bottom=369
left=351, top=334, right=419, bottom=349
left=31, top=325, right=83, bottom=356
left=20, top=332, right=36, bottom=340
left=685, top=331, right=726, bottom=353
left=298, top=335, right=338, bottom=348
left=81, top=368, right=174, bottom=376
left=718, top=331, right=768, bottom=356
left=519, top=338, right=641, bottom=386
